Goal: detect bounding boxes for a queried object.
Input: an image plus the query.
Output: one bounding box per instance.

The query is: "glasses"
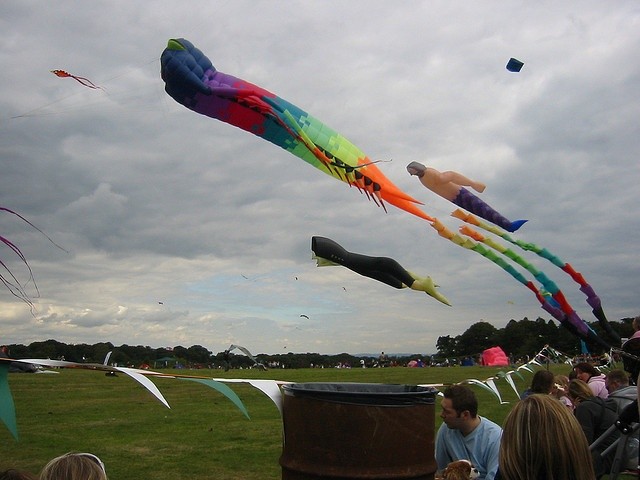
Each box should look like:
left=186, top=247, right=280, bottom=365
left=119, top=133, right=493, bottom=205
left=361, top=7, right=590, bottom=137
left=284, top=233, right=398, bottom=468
left=604, top=381, right=615, bottom=390
left=444, top=386, right=452, bottom=399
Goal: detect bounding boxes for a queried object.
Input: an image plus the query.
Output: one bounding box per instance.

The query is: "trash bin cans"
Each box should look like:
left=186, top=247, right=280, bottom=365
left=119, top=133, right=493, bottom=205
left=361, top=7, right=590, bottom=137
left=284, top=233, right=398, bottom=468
left=278, top=382, right=440, bottom=480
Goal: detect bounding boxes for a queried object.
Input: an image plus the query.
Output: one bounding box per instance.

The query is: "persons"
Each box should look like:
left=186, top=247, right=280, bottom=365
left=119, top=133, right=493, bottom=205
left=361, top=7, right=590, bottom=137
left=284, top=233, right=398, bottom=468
left=520, top=371, right=554, bottom=400
left=622, top=315, right=640, bottom=385
left=434, top=386, right=504, bottom=479
left=498, top=394, right=595, bottom=480
left=602, top=370, right=638, bottom=411
left=0, top=468, right=36, bottom=480
left=568, top=380, right=618, bottom=447
left=573, top=363, right=609, bottom=399
left=552, top=374, right=574, bottom=412
left=39, top=452, right=108, bottom=480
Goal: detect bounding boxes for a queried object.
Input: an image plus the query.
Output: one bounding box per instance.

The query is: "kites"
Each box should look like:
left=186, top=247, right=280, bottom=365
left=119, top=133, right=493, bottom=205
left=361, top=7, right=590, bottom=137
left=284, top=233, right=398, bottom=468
left=311, top=236, right=452, bottom=307
left=448, top=208, right=621, bottom=346
left=228, top=345, right=259, bottom=365
left=162, top=38, right=436, bottom=223
left=457, top=225, right=609, bottom=351
left=407, top=161, right=528, bottom=233
left=51, top=69, right=105, bottom=90
left=428, top=218, right=582, bottom=340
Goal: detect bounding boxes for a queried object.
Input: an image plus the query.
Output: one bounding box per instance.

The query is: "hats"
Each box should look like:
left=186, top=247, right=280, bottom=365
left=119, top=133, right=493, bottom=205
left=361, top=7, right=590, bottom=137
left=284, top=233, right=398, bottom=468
left=611, top=337, right=639, bottom=361
